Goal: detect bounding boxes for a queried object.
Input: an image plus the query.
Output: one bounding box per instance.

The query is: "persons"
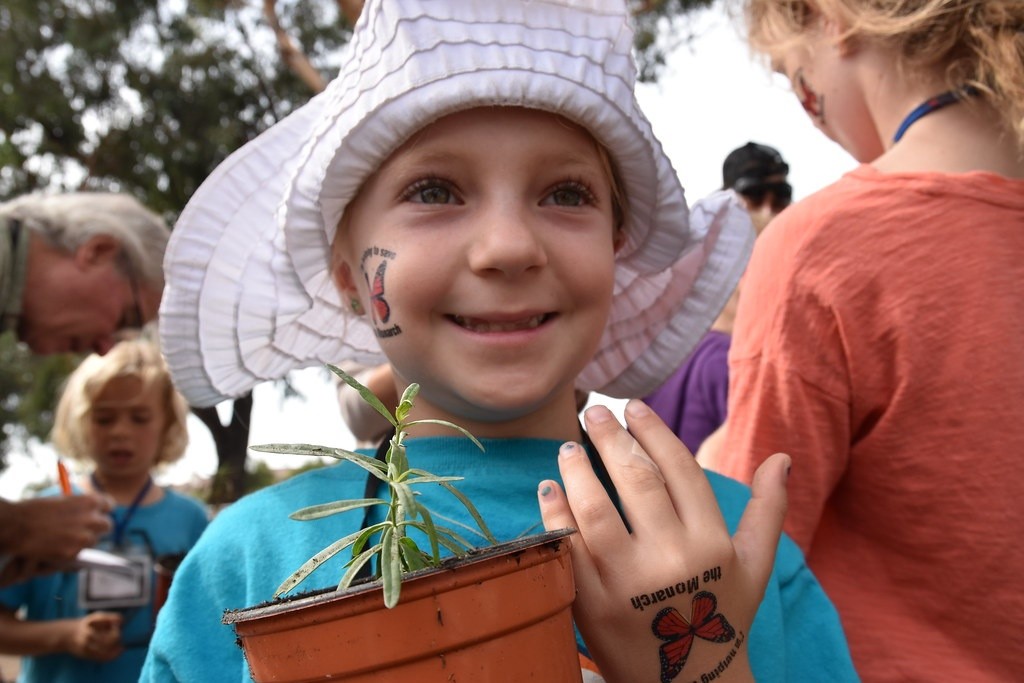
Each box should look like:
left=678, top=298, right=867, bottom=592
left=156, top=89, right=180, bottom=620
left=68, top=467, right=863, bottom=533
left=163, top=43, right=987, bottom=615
left=626, top=0, right=1024, bottom=683
left=2, top=192, right=169, bottom=581
left=138, top=0, right=864, bottom=683
left=0, top=339, right=211, bottom=682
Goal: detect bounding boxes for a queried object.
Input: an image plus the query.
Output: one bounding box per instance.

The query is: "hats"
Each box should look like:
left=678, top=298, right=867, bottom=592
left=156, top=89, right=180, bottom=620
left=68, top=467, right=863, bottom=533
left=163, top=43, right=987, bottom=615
left=159, top=0, right=754, bottom=408
left=722, top=141, right=790, bottom=184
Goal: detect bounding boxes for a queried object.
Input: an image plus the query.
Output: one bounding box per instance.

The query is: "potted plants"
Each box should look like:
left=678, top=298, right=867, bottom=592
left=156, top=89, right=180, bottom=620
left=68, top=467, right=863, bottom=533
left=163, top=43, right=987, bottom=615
left=222, top=361, right=583, bottom=682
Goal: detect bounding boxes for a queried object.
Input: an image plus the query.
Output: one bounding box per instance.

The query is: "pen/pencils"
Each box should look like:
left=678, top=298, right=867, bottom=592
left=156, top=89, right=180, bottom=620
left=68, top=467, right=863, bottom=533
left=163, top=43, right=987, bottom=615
left=56, top=458, right=70, bottom=497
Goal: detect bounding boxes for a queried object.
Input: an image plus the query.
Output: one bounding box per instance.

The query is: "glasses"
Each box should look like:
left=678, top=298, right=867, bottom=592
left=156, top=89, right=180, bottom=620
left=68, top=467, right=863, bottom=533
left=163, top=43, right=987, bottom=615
left=118, top=254, right=144, bottom=339
left=739, top=185, right=794, bottom=208
